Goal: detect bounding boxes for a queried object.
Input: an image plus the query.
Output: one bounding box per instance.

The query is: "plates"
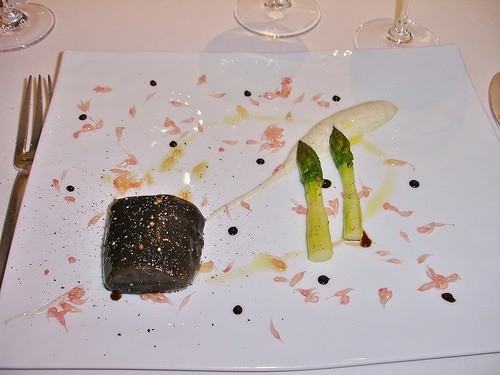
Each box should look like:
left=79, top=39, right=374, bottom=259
left=0, top=43, right=500, bottom=375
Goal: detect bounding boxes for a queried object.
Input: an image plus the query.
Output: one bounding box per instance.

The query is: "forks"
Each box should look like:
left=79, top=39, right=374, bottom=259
left=0, top=74, right=57, bottom=289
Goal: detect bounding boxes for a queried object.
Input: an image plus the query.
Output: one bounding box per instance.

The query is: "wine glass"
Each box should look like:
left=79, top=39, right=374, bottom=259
left=1, top=1, right=55, bottom=53
left=354, top=1, right=440, bottom=49
left=233, top=0, right=322, bottom=38
